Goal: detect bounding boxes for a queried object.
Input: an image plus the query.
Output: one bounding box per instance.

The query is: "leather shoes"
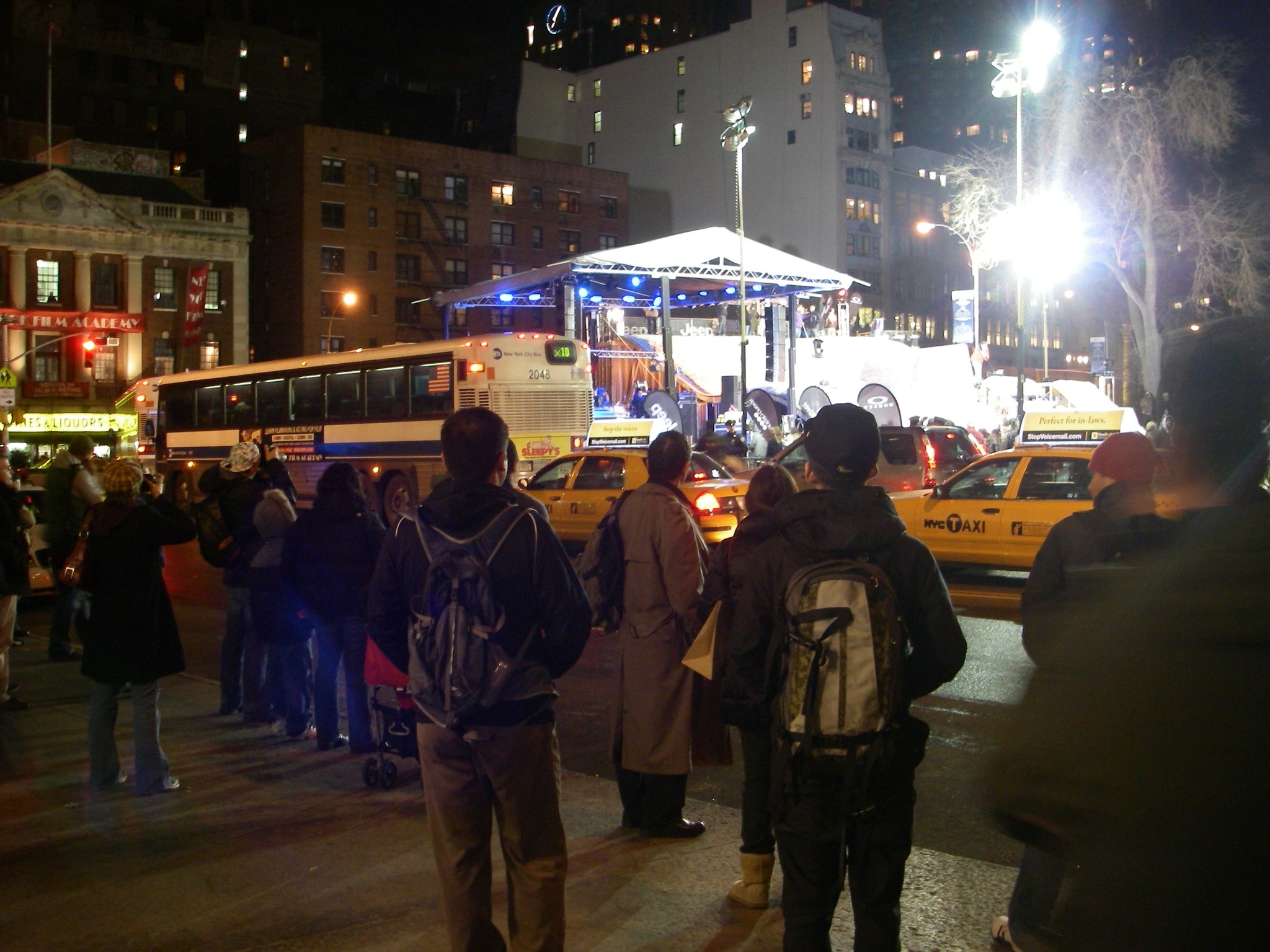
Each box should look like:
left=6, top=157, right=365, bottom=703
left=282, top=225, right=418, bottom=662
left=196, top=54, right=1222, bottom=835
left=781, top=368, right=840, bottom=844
left=649, top=818, right=706, bottom=837
left=621, top=809, right=647, bottom=828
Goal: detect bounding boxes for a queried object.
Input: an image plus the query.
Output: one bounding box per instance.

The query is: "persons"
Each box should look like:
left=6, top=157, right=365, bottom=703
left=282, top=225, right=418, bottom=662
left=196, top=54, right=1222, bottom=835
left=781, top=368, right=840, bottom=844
left=969, top=316, right=1270, bottom=952
left=0, top=390, right=801, bottom=952
left=723, top=401, right=968, bottom=952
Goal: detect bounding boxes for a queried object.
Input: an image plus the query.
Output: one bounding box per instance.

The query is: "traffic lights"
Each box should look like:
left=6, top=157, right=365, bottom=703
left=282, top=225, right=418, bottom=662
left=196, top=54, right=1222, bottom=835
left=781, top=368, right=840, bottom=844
left=82, top=340, right=96, bottom=370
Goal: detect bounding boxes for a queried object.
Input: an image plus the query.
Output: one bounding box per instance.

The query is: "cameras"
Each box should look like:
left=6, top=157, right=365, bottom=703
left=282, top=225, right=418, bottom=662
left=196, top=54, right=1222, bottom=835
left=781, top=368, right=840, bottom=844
left=257, top=443, right=287, bottom=465
left=139, top=474, right=155, bottom=493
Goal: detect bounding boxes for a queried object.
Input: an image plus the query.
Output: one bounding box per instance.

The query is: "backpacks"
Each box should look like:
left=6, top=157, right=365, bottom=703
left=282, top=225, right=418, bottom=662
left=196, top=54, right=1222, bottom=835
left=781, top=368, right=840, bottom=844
left=397, top=504, right=548, bottom=726
left=767, top=514, right=906, bottom=765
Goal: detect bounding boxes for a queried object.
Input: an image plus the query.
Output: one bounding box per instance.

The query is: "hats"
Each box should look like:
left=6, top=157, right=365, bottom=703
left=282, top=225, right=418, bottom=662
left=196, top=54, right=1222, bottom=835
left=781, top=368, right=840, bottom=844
left=70, top=436, right=94, bottom=456
left=803, top=403, right=878, bottom=484
left=724, top=420, right=736, bottom=426
left=102, top=460, right=143, bottom=495
left=219, top=442, right=259, bottom=472
left=1088, top=432, right=1156, bottom=485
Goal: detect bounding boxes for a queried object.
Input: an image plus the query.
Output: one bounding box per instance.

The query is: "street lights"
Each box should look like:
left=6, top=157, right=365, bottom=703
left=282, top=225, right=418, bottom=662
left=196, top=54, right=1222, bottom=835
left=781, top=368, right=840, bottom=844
left=916, top=221, right=981, bottom=385
left=720, top=96, right=756, bottom=442
left=327, top=292, right=356, bottom=352
left=990, top=51, right=1049, bottom=425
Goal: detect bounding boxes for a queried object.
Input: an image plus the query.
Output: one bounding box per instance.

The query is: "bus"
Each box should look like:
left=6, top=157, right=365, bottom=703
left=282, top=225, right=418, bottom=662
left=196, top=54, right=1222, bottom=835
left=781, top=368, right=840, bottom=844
left=0, top=428, right=123, bottom=488
left=114, top=329, right=595, bottom=509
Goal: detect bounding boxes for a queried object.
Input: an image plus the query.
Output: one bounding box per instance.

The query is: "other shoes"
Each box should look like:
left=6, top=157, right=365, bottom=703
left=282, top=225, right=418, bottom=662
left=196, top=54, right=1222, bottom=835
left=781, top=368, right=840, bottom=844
left=50, top=648, right=84, bottom=661
left=218, top=700, right=381, bottom=758
left=165, top=778, right=180, bottom=790
left=3, top=696, right=31, bottom=713
left=16, top=628, right=30, bottom=637
left=6, top=683, right=19, bottom=692
left=116, top=772, right=127, bottom=783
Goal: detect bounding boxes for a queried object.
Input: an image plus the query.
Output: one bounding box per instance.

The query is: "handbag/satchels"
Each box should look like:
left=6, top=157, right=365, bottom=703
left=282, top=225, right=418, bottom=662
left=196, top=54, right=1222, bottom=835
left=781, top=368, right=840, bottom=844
left=59, top=543, right=86, bottom=586
left=590, top=489, right=634, bottom=632
left=682, top=601, right=720, bottom=680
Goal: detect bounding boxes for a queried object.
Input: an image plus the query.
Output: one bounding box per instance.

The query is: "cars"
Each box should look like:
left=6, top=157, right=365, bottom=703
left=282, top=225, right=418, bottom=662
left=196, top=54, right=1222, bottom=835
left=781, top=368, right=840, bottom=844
left=512, top=418, right=750, bottom=551
left=734, top=421, right=987, bottom=494
left=887, top=405, right=1139, bottom=570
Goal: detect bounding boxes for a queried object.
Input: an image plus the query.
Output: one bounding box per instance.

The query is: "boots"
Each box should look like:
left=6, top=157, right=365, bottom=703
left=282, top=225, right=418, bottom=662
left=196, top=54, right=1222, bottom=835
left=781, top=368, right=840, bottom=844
left=729, top=844, right=776, bottom=908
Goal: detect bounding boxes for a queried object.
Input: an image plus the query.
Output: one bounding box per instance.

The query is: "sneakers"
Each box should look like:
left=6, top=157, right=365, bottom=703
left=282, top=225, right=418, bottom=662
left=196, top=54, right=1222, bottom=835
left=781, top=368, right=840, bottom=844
left=993, top=916, right=1023, bottom=952
